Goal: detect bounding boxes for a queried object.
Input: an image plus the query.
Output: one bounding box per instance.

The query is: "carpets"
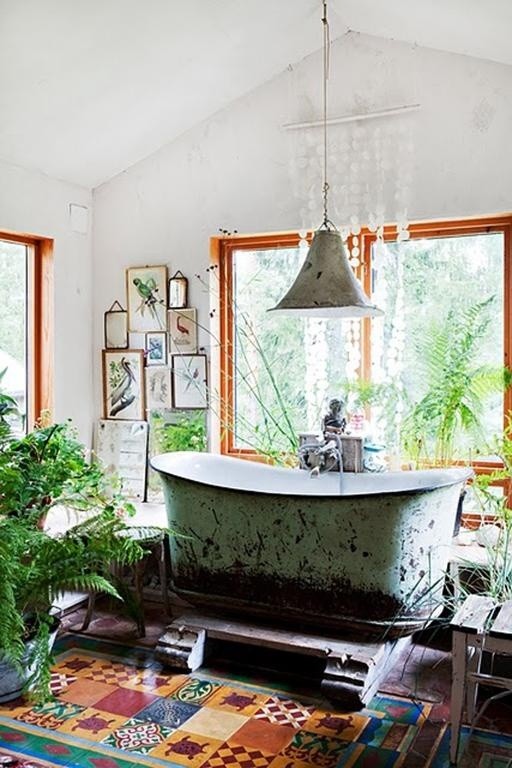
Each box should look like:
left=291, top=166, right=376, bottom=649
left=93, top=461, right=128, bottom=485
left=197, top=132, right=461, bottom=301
left=0, top=633, right=435, bottom=768
left=426, top=721, right=511, bottom=766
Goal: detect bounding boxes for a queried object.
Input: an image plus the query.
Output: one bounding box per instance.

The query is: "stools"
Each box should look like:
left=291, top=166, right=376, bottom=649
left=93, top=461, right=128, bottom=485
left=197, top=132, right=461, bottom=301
left=447, top=593, right=511, bottom=768
left=78, top=525, right=172, bottom=639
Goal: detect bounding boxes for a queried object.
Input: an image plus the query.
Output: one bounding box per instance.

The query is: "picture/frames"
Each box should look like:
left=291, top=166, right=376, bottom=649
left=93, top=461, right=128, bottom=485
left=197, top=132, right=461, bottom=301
left=102, top=264, right=209, bottom=422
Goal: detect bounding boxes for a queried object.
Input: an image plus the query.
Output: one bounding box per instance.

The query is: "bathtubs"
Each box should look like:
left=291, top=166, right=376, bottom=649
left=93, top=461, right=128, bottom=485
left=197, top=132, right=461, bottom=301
left=150, top=451, right=475, bottom=640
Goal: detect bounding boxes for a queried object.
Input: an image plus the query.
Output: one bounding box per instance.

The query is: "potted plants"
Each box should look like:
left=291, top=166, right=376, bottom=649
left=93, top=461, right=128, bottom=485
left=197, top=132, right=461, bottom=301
left=1, top=366, right=196, bottom=705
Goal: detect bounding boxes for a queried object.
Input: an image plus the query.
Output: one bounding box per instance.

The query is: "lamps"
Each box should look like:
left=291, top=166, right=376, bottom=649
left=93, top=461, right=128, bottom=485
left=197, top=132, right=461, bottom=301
left=265, top=0, right=385, bottom=320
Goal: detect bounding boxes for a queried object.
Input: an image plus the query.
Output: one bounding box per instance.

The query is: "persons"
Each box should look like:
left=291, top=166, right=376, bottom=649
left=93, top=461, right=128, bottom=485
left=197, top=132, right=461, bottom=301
left=310, top=398, right=347, bottom=477
